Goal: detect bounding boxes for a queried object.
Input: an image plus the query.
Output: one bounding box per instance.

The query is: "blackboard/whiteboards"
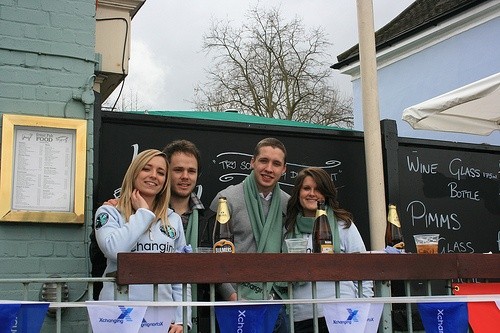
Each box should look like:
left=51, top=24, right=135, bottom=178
left=398, top=137, right=497, bottom=333
left=96, top=110, right=394, bottom=333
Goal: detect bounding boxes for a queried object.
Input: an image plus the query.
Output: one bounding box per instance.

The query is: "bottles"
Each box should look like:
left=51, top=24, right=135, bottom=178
left=212, top=197, right=235, bottom=253
left=384, top=204, right=406, bottom=254
left=311, top=199, right=333, bottom=253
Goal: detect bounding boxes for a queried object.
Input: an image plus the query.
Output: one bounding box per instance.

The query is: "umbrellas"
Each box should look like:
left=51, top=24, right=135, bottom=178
left=402, top=73, right=500, bottom=136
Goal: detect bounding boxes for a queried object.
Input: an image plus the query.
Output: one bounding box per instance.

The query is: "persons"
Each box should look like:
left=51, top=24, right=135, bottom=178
left=208, top=137, right=291, bottom=333
left=95, top=149, right=192, bottom=333
left=280, top=168, right=373, bottom=333
left=103, top=141, right=219, bottom=333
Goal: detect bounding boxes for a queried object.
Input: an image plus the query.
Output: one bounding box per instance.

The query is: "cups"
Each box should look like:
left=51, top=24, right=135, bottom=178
left=284, top=238, right=309, bottom=253
left=413, top=234, right=440, bottom=254
